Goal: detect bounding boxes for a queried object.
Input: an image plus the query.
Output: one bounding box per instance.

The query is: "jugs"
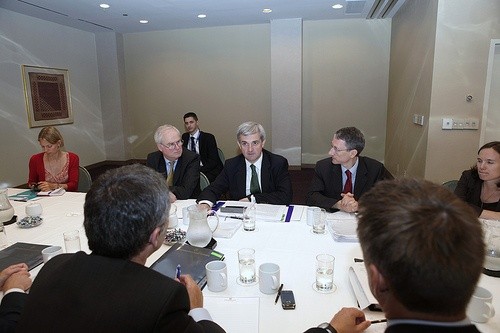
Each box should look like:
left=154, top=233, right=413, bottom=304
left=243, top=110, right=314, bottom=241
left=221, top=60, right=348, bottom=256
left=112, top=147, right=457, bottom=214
left=0, top=188, right=15, bottom=223
left=187, top=204, right=220, bottom=247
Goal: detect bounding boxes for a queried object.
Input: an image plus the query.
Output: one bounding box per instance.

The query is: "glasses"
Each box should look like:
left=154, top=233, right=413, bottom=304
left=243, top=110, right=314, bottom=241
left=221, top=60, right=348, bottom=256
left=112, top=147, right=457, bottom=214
left=328, top=143, right=348, bottom=152
left=161, top=139, right=184, bottom=150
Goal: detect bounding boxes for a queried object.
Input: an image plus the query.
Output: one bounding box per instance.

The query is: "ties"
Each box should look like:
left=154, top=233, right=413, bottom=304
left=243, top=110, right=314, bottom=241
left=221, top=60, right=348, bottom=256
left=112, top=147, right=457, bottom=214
left=165, top=161, right=175, bottom=187
left=190, top=135, right=196, bottom=153
left=344, top=169, right=353, bottom=194
left=249, top=164, right=262, bottom=195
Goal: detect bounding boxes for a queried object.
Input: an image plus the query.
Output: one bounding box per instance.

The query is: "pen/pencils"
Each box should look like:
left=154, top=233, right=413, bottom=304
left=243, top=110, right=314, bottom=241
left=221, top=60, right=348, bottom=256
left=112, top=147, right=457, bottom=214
left=370, top=319, right=387, bottom=324
left=48, top=189, right=55, bottom=194
left=175, top=264, right=182, bottom=279
left=280, top=213, right=285, bottom=222
left=14, top=199, right=27, bottom=202
left=275, top=283, right=284, bottom=305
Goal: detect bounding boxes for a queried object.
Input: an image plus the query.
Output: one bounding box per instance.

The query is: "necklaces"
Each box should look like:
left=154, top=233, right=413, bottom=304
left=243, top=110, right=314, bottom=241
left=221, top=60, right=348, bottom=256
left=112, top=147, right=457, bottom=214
left=481, top=184, right=498, bottom=202
left=47, top=154, right=62, bottom=186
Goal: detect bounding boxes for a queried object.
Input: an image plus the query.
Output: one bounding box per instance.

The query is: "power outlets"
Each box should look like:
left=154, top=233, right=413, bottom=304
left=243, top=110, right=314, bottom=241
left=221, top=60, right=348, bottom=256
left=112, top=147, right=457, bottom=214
left=452, top=118, right=479, bottom=130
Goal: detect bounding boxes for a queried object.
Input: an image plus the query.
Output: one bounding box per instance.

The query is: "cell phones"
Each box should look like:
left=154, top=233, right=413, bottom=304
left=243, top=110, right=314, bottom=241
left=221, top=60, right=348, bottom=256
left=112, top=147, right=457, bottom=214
left=280, top=291, right=295, bottom=309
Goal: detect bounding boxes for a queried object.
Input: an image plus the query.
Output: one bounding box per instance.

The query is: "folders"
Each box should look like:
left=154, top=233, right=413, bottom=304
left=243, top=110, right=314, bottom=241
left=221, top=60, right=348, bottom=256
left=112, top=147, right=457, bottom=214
left=0, top=241, right=50, bottom=272
left=149, top=242, right=226, bottom=292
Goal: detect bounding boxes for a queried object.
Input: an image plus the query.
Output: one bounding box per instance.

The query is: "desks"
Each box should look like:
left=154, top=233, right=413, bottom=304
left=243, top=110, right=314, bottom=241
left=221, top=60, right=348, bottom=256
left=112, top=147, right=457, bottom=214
left=0, top=187, right=500, bottom=333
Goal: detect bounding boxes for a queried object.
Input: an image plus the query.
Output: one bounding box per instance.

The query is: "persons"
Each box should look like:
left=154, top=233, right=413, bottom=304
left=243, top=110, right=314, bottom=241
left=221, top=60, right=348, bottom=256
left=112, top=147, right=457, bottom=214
left=196, top=121, right=292, bottom=216
left=181, top=112, right=224, bottom=184
left=144, top=123, right=198, bottom=202
left=302, top=177, right=482, bottom=333
left=20, top=163, right=226, bottom=333
left=306, top=126, right=396, bottom=213
left=26, top=125, right=80, bottom=194
left=0, top=262, right=32, bottom=333
left=454, top=140, right=500, bottom=220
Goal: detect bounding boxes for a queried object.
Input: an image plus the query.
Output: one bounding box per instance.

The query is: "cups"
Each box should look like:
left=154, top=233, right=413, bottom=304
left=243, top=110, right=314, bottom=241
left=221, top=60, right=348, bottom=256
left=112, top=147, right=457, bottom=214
left=258, top=262, right=280, bottom=295
left=205, top=260, right=227, bottom=292
left=466, top=286, right=496, bottom=323
left=238, top=249, right=257, bottom=283
left=307, top=207, right=321, bottom=226
left=314, top=254, right=336, bottom=292
left=41, top=245, right=63, bottom=265
left=242, top=206, right=256, bottom=232
left=167, top=202, right=177, bottom=228
left=313, top=207, right=327, bottom=234
left=63, top=230, right=80, bottom=253
left=478, top=217, right=500, bottom=272
left=182, top=208, right=190, bottom=225
left=25, top=203, right=42, bottom=217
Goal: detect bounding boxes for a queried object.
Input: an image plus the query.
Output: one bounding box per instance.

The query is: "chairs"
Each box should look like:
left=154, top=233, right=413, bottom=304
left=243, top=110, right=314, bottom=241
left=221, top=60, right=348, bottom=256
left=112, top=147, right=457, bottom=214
left=200, top=147, right=226, bottom=191
left=77, top=166, right=92, bottom=193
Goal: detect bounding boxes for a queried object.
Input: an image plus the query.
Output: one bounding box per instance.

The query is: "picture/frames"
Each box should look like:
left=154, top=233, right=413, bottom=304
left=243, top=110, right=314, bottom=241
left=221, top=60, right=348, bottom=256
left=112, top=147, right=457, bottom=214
left=21, top=64, right=75, bottom=129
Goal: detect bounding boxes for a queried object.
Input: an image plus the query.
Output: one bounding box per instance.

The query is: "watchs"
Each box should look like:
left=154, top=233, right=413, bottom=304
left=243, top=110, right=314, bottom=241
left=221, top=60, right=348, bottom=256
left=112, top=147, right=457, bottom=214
left=318, top=322, right=338, bottom=333
